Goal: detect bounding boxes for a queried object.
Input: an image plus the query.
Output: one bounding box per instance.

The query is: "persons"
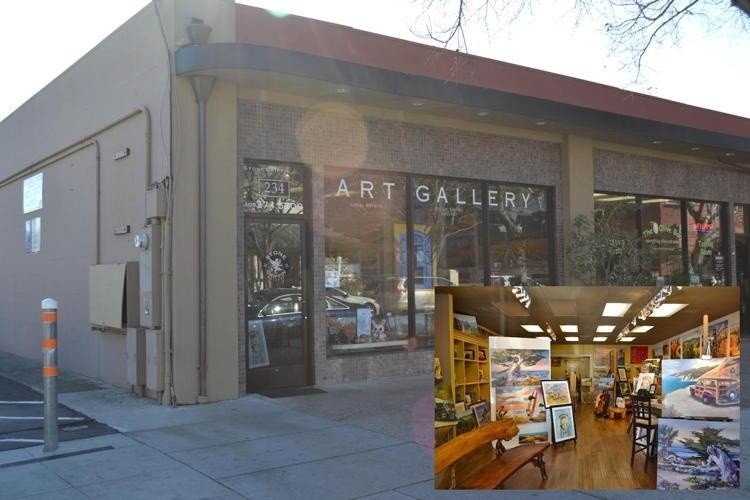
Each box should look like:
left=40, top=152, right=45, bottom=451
left=371, top=319, right=387, bottom=342
left=560, top=414, right=571, bottom=438
left=706, top=445, right=739, bottom=486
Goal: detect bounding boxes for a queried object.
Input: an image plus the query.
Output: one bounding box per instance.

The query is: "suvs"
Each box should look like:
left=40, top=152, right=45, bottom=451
left=689, top=378, right=740, bottom=406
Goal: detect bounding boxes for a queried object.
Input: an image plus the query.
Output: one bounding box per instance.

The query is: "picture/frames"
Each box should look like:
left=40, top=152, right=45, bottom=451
left=649, top=384, right=656, bottom=395
left=468, top=399, right=491, bottom=426
left=617, top=367, right=632, bottom=397
left=540, top=379, right=577, bottom=445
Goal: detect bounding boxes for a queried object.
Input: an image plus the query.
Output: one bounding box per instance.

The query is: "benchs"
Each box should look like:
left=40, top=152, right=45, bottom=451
left=434, top=417, right=550, bottom=490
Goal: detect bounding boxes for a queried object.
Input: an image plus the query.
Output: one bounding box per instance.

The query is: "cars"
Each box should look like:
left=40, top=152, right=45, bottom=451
left=386, top=276, right=455, bottom=312
left=247, top=287, right=380, bottom=319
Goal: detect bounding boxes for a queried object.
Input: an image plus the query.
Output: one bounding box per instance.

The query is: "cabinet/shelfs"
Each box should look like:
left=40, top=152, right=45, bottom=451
left=435, top=293, right=489, bottom=418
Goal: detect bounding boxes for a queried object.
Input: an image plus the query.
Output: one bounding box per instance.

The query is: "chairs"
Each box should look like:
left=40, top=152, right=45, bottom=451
left=629, top=394, right=658, bottom=474
